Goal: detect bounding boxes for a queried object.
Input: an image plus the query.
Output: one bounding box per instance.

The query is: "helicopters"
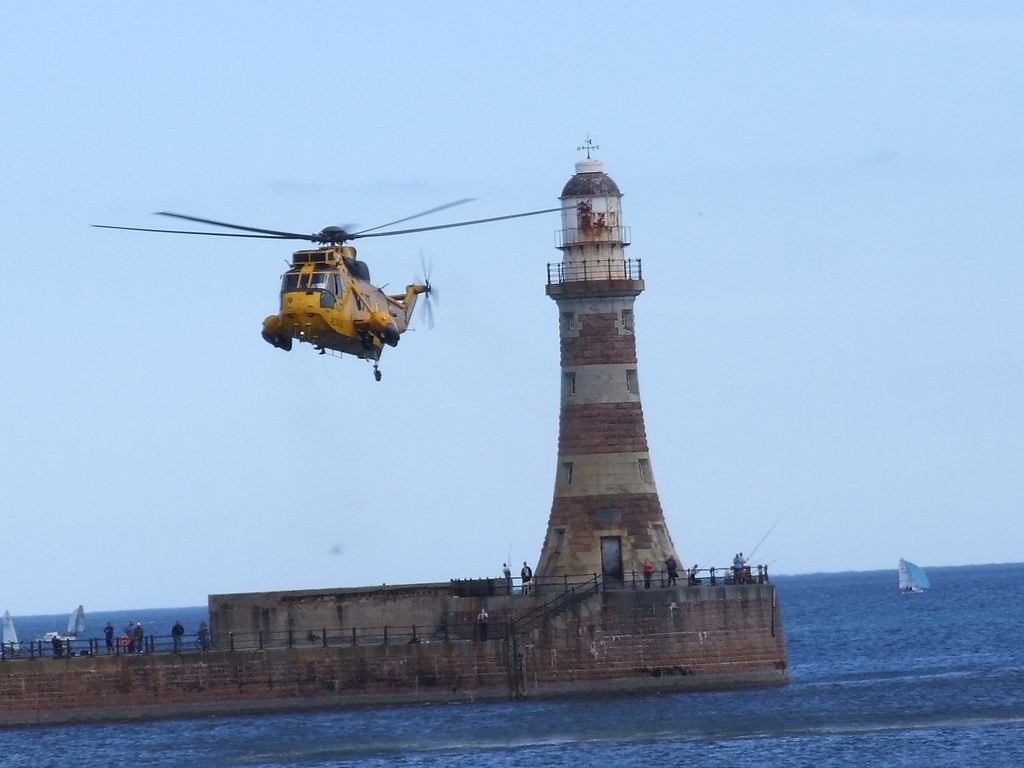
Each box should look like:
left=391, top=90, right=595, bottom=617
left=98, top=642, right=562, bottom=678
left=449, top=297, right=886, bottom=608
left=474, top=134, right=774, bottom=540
left=85, top=191, right=591, bottom=383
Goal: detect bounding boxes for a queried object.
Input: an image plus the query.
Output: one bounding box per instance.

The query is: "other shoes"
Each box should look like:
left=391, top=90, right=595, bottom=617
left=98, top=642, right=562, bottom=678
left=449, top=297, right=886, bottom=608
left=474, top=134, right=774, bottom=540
left=314, top=347, right=320, bottom=350
left=319, top=350, right=325, bottom=354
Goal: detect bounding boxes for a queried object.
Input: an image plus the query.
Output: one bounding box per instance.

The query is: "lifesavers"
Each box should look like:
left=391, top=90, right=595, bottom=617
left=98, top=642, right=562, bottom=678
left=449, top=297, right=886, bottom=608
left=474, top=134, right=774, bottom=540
left=120, top=635, right=132, bottom=647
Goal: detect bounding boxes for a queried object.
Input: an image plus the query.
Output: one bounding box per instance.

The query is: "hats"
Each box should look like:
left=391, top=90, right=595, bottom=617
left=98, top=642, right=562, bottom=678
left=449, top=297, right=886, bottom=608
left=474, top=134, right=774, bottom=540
left=137, top=623, right=140, bottom=626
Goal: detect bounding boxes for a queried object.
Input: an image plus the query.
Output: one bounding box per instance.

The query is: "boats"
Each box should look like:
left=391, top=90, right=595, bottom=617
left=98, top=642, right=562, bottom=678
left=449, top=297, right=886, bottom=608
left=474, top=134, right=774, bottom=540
left=36, top=631, right=62, bottom=643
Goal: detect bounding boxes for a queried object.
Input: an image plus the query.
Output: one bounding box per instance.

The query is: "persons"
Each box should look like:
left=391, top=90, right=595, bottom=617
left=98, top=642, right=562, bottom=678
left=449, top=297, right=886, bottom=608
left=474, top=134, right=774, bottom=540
left=199, top=621, right=209, bottom=651
left=52, top=636, right=59, bottom=655
left=733, top=553, right=746, bottom=582
left=503, top=563, right=513, bottom=593
left=124, top=620, right=143, bottom=653
left=104, top=622, right=115, bottom=655
left=691, top=565, right=698, bottom=581
left=292, top=275, right=307, bottom=288
left=477, top=609, right=488, bottom=641
left=665, top=555, right=679, bottom=587
left=642, top=558, right=654, bottom=589
left=521, top=562, right=532, bottom=594
left=172, top=620, right=184, bottom=653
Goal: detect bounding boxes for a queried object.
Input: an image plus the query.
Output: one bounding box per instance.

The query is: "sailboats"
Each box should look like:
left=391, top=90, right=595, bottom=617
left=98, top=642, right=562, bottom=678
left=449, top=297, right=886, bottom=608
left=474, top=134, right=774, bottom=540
left=2, top=610, right=21, bottom=652
left=60, top=604, right=88, bottom=641
left=898, top=557, right=931, bottom=594
